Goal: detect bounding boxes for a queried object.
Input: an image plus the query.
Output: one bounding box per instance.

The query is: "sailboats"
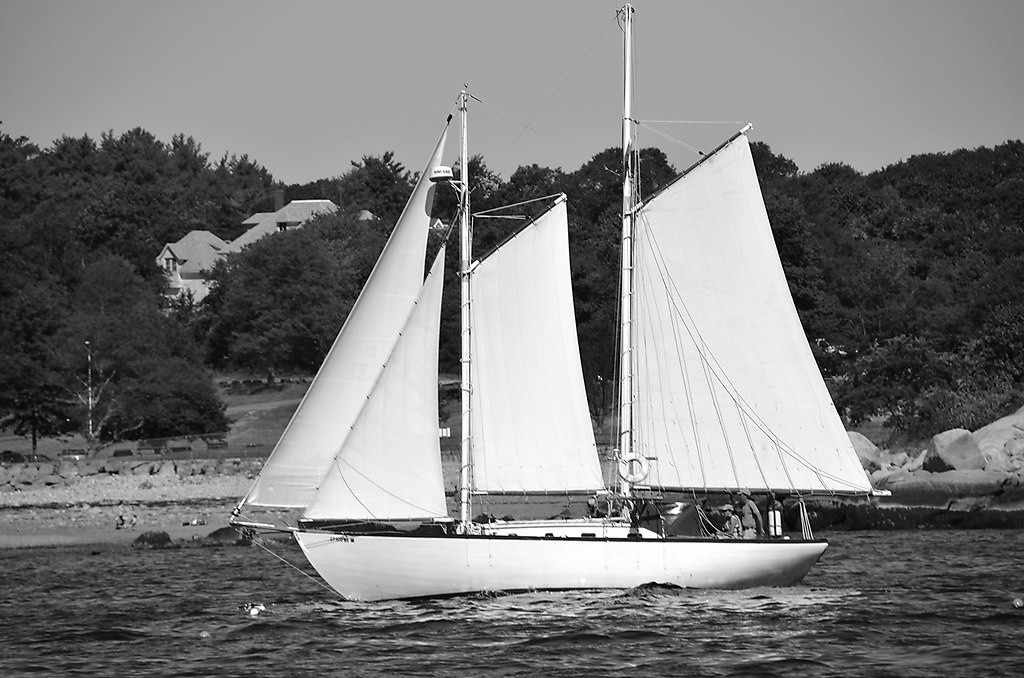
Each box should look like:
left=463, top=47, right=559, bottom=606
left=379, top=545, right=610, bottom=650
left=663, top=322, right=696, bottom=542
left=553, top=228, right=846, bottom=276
left=227, top=5, right=896, bottom=605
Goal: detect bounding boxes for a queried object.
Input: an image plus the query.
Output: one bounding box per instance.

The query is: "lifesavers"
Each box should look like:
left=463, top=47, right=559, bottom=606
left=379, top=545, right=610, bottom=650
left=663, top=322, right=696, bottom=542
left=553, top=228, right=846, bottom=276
left=618, top=452, right=649, bottom=483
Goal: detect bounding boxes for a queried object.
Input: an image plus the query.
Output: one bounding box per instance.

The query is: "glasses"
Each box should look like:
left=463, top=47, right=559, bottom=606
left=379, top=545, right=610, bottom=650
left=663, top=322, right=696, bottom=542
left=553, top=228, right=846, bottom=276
left=720, top=510, right=729, bottom=513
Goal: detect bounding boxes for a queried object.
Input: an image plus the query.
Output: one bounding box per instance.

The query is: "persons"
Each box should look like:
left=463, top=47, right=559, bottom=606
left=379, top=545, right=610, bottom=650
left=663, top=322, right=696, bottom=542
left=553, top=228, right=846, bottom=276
left=737, top=489, right=765, bottom=539
left=718, top=503, right=742, bottom=539
left=130, top=515, right=138, bottom=531
left=116, top=516, right=126, bottom=529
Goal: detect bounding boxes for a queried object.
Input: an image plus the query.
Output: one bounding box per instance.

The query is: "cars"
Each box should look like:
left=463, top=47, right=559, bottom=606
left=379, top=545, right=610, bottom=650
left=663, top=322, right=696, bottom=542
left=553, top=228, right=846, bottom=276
left=0, top=450, right=52, bottom=466
left=56, top=448, right=88, bottom=464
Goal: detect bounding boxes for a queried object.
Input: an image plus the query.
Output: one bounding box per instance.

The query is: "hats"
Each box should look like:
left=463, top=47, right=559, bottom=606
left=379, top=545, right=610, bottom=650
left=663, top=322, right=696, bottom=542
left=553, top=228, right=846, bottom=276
left=737, top=489, right=751, bottom=497
left=718, top=504, right=734, bottom=511
